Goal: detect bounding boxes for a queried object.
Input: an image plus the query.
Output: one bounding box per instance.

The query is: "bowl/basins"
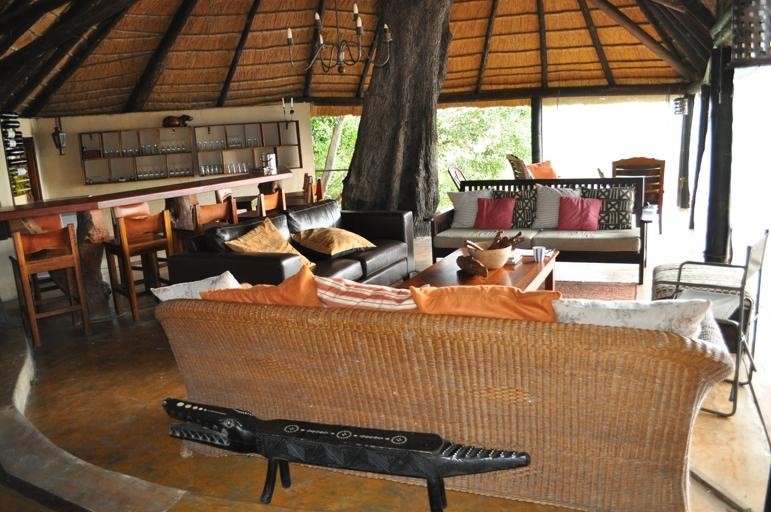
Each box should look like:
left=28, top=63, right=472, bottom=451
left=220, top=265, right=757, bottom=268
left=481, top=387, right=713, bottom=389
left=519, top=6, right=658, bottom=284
left=465, top=240, right=511, bottom=269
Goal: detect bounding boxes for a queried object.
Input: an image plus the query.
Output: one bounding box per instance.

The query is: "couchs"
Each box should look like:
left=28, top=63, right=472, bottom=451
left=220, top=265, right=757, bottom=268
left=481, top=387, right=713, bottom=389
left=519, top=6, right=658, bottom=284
left=153, top=297, right=738, bottom=511
left=168, top=198, right=417, bottom=289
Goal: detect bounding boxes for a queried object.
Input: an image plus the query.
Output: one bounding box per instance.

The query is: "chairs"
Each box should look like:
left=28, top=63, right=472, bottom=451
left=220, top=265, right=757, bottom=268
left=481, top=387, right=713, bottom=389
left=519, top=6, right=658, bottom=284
left=428, top=154, right=666, bottom=286
left=669, top=230, right=769, bottom=420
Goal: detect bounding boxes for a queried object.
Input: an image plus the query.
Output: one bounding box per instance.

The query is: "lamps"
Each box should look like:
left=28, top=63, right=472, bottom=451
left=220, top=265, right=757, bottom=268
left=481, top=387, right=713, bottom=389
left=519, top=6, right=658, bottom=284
left=673, top=88, right=694, bottom=119
left=728, top=2, right=771, bottom=70
left=285, top=2, right=397, bottom=74
left=52, top=119, right=70, bottom=158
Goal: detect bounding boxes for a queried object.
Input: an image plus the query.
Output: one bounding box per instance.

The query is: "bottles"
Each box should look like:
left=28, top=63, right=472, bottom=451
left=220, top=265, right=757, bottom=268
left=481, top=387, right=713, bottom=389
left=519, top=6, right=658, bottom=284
left=103, top=136, right=277, bottom=182
left=0, top=111, right=32, bottom=197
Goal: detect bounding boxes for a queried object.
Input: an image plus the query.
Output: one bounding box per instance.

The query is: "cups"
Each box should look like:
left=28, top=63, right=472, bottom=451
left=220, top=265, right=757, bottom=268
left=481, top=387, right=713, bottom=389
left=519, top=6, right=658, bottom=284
left=532, top=246, right=545, bottom=262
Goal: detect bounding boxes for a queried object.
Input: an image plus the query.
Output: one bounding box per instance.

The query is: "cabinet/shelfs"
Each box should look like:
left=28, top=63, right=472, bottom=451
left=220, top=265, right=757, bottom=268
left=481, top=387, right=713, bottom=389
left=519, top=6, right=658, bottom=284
left=1, top=110, right=46, bottom=207
left=75, top=120, right=312, bottom=186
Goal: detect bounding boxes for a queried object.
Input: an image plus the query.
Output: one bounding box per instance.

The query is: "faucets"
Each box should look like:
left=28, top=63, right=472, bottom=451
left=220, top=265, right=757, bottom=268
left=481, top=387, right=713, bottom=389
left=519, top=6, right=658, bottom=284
left=260, top=154, right=272, bottom=167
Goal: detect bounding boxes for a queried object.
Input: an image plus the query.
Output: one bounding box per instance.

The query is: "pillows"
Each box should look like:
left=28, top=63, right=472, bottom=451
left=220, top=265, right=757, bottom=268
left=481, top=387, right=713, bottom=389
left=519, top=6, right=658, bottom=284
left=223, top=216, right=378, bottom=271
